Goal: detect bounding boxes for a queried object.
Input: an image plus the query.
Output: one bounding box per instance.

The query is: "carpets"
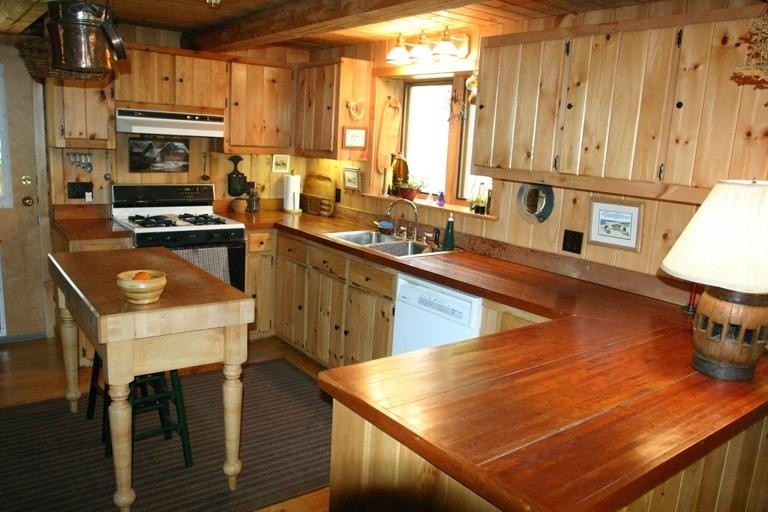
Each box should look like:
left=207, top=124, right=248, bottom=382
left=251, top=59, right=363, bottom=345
left=0, top=358, right=332, bottom=512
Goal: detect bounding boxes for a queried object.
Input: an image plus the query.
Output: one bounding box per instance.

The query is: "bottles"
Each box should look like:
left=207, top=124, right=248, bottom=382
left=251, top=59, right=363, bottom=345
left=438, top=190, right=444, bottom=206
left=475, top=183, right=485, bottom=215
left=486, top=184, right=493, bottom=213
left=441, top=211, right=454, bottom=251
left=470, top=183, right=476, bottom=213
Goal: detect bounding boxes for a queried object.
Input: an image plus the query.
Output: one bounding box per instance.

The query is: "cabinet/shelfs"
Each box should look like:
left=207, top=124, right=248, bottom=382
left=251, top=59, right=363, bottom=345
left=661, top=15, right=768, bottom=191
left=54, top=233, right=133, bottom=366
left=227, top=59, right=296, bottom=150
left=305, top=235, right=397, bottom=369
left=114, top=45, right=228, bottom=112
left=318, top=317, right=768, bottom=512
left=472, top=36, right=568, bottom=175
left=292, top=57, right=372, bottom=160
left=555, top=22, right=679, bottom=186
left=245, top=229, right=276, bottom=341
left=481, top=298, right=552, bottom=335
left=274, top=227, right=307, bottom=356
left=45, top=80, right=116, bottom=220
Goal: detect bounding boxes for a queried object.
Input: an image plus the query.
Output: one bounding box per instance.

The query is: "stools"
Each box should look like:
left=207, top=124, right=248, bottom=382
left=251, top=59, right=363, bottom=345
left=87, top=350, right=165, bottom=441
left=106, top=370, right=193, bottom=468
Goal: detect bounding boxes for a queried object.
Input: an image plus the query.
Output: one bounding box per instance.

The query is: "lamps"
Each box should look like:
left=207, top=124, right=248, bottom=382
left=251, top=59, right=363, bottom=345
left=659, top=178, right=768, bottom=380
left=385, top=34, right=410, bottom=61
left=433, top=32, right=458, bottom=55
left=408, top=34, right=434, bottom=59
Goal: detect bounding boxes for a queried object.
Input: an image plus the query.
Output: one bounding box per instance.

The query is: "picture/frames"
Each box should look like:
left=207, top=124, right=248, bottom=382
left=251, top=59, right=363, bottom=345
left=343, top=168, right=360, bottom=190
left=129, top=137, right=190, bottom=173
left=344, top=127, right=367, bottom=148
left=273, top=155, right=290, bottom=172
left=587, top=197, right=645, bottom=254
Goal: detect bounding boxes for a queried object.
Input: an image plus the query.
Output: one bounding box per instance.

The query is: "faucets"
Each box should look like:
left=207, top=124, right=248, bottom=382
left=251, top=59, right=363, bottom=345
left=384, top=197, right=420, bottom=241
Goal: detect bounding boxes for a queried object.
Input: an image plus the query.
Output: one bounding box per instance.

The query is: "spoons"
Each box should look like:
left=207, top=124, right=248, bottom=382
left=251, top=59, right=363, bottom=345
left=201, top=152, right=210, bottom=181
left=103, top=151, right=112, bottom=181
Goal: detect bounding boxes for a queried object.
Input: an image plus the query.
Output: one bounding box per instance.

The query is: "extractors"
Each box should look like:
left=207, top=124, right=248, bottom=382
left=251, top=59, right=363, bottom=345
left=115, top=108, right=225, bottom=139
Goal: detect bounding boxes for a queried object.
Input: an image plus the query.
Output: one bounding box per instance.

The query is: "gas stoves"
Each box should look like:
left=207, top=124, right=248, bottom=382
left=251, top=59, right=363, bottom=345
left=112, top=206, right=247, bottom=249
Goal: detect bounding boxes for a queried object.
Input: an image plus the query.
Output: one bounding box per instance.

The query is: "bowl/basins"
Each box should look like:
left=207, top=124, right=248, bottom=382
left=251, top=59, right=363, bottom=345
left=115, top=269, right=167, bottom=305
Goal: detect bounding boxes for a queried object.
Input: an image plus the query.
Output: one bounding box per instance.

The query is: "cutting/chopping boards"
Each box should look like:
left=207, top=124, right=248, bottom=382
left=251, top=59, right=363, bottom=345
left=300, top=172, right=336, bottom=218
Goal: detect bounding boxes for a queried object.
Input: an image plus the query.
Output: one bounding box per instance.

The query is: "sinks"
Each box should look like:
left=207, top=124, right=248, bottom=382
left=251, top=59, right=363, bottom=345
left=322, top=227, right=464, bottom=260
